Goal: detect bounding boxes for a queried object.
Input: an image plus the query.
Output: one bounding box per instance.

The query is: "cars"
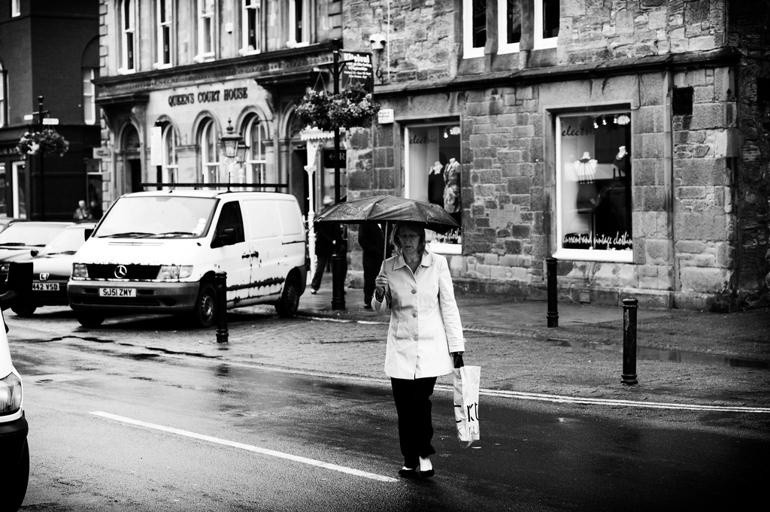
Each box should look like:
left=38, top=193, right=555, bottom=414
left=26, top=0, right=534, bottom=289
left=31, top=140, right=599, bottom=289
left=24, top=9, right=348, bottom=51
left=11, top=221, right=97, bottom=318
left=0, top=309, right=31, bottom=511
left=0, top=221, right=76, bottom=301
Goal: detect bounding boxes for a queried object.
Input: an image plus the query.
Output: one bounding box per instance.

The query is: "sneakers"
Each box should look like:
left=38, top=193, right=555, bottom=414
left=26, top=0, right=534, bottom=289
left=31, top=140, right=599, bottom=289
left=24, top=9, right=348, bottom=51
left=364, top=303, right=371, bottom=308
left=311, top=288, right=316, bottom=294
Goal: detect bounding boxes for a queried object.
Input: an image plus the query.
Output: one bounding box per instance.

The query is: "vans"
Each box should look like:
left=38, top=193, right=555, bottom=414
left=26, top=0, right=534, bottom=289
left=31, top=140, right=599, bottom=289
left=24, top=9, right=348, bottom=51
left=64, top=191, right=307, bottom=327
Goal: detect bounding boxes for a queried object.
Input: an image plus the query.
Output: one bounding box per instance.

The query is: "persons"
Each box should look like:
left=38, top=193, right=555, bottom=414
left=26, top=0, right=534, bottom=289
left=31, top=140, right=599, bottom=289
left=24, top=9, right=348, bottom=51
left=310, top=223, right=347, bottom=294
left=371, top=221, right=464, bottom=477
left=359, top=223, right=394, bottom=309
left=574, top=152, right=598, bottom=209
left=610, top=145, right=629, bottom=207
left=443, top=157, right=461, bottom=214
left=428, top=161, right=445, bottom=209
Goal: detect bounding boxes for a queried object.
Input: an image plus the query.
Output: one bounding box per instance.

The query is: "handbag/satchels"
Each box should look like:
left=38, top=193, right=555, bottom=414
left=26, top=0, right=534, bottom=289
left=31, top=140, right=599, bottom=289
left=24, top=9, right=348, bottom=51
left=451, top=353, right=480, bottom=442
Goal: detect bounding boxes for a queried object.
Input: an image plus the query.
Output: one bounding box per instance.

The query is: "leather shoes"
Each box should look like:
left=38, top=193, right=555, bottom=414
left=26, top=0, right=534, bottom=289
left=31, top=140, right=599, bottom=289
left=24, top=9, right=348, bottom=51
left=398, top=465, right=434, bottom=477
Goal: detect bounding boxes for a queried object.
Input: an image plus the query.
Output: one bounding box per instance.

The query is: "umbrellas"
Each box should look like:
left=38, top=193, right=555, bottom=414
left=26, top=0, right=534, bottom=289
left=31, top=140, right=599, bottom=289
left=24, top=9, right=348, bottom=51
left=313, top=194, right=461, bottom=275
